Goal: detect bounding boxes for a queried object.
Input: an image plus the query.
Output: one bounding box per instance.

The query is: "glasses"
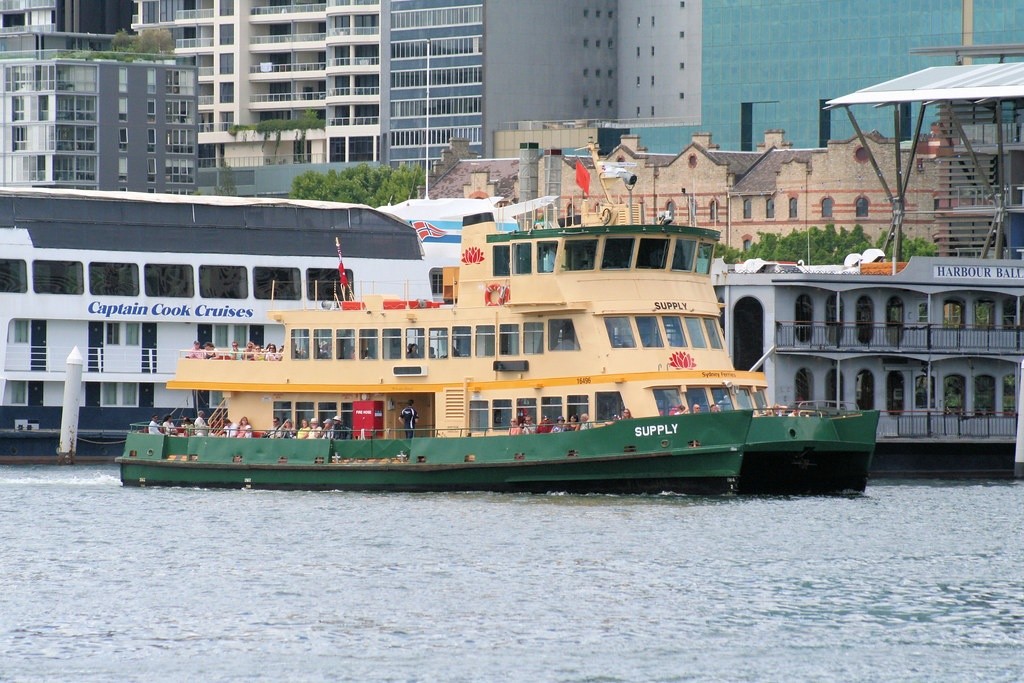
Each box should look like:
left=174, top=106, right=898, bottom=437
left=233, top=345, right=237, bottom=347
left=273, top=421, right=278, bottom=423
left=511, top=421, right=515, bottom=423
left=269, top=347, right=275, bottom=349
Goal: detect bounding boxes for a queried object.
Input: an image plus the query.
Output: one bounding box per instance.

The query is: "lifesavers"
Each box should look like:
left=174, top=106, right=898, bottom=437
left=485, top=284, right=504, bottom=306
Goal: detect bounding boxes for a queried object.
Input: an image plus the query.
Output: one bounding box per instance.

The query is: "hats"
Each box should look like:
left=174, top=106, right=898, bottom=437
left=557, top=416, right=564, bottom=421
left=323, top=419, right=333, bottom=426
left=194, top=341, right=200, bottom=345
left=309, top=418, right=319, bottom=424
left=333, top=416, right=342, bottom=422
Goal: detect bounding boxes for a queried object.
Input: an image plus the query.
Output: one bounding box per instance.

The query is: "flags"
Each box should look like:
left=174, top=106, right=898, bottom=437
left=575, top=158, right=590, bottom=195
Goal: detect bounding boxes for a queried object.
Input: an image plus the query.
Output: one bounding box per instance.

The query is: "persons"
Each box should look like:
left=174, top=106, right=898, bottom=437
left=345, top=346, right=372, bottom=360
left=694, top=404, right=700, bottom=413
left=605, top=410, right=631, bottom=425
left=407, top=343, right=423, bottom=358
left=543, top=243, right=556, bottom=272
left=508, top=414, right=593, bottom=435
left=764, top=405, right=799, bottom=417
left=264, top=416, right=352, bottom=439
left=430, top=347, right=436, bottom=358
left=319, top=342, right=332, bottom=358
left=186, top=341, right=219, bottom=360
left=710, top=405, right=719, bottom=412
left=399, top=400, right=418, bottom=438
left=183, top=411, right=208, bottom=436
left=149, top=415, right=178, bottom=436
left=295, top=344, right=306, bottom=359
left=674, top=406, right=689, bottom=415
left=230, top=342, right=284, bottom=361
left=211, top=413, right=252, bottom=438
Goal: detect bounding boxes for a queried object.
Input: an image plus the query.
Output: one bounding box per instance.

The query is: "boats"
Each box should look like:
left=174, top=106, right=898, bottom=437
left=115, top=134, right=883, bottom=504
